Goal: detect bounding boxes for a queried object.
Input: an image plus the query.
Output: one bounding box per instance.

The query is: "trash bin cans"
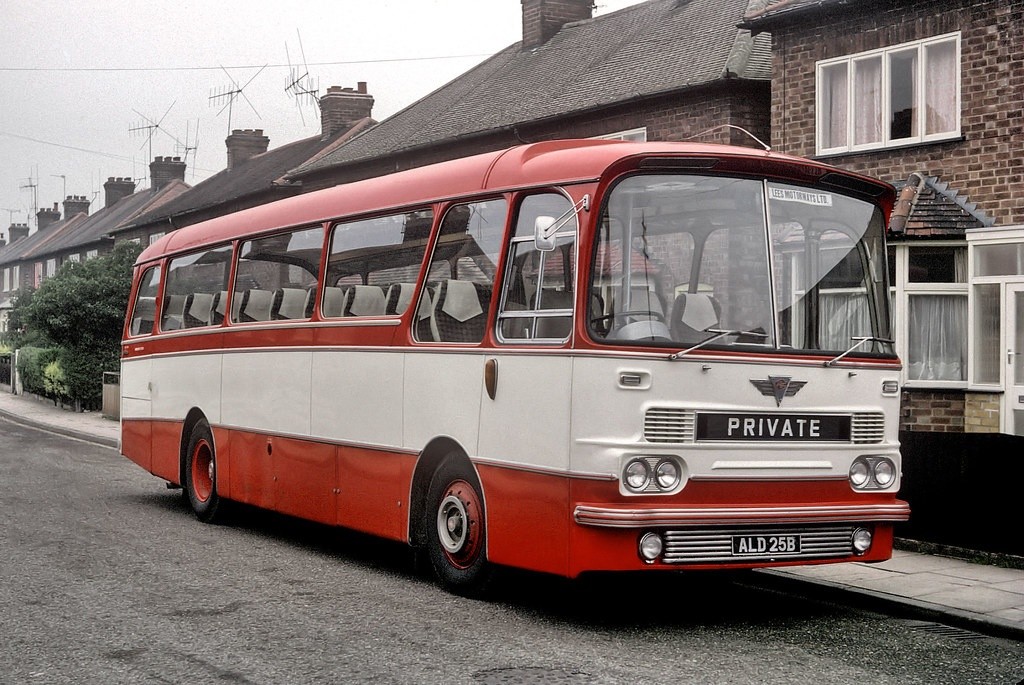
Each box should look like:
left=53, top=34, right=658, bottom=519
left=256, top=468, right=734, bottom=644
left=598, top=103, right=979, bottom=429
left=101, top=372, right=120, bottom=421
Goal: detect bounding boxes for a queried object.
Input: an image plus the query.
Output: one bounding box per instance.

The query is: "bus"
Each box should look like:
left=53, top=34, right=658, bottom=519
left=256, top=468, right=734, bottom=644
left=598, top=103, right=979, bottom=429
left=119, top=125, right=911, bottom=596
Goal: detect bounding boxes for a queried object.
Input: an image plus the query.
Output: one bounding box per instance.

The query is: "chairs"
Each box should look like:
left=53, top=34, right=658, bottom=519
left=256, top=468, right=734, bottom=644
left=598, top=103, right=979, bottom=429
left=130, top=265, right=727, bottom=350
